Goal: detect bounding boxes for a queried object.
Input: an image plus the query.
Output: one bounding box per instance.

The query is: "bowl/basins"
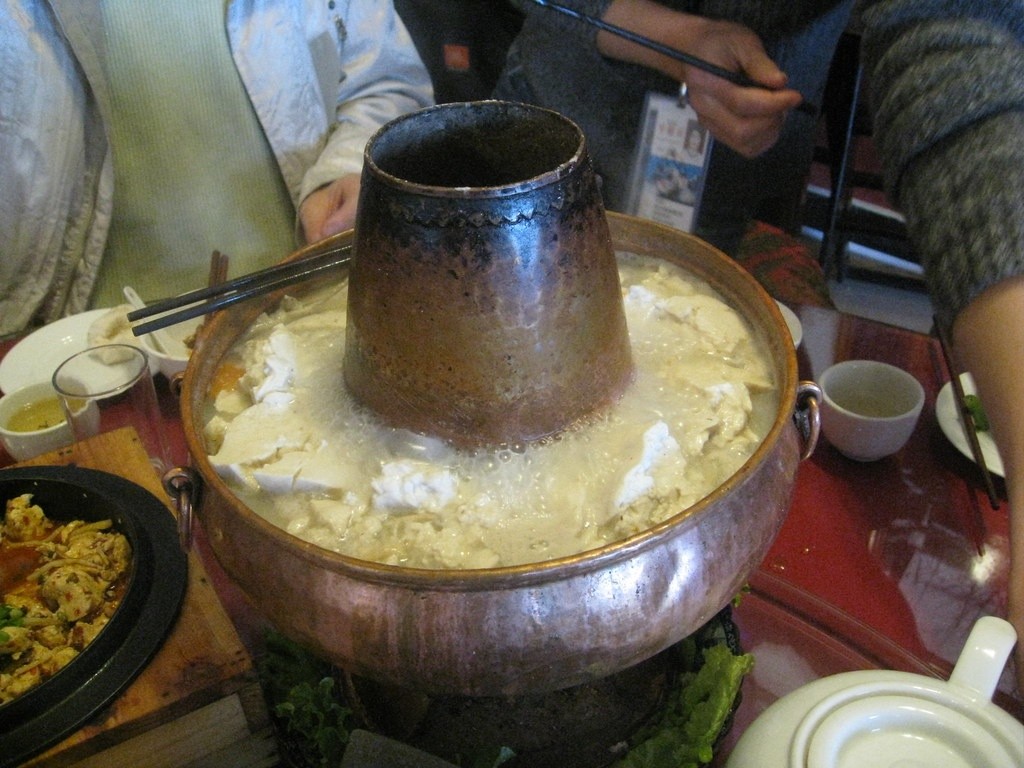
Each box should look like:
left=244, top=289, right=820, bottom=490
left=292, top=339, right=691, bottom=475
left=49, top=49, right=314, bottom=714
left=0, top=379, right=102, bottom=464
left=135, top=291, right=248, bottom=379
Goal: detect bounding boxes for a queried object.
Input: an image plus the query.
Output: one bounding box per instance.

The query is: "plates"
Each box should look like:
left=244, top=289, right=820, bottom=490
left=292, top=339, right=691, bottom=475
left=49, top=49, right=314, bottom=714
left=934, top=370, right=1007, bottom=480
left=0, top=464, right=191, bottom=768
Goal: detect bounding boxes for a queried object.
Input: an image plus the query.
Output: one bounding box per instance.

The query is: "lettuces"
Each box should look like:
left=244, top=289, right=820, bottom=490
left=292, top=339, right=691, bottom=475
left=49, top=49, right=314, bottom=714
left=248, top=621, right=753, bottom=768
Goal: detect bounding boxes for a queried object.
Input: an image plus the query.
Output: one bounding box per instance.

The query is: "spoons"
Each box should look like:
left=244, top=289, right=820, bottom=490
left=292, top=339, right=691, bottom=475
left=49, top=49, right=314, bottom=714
left=122, top=285, right=188, bottom=360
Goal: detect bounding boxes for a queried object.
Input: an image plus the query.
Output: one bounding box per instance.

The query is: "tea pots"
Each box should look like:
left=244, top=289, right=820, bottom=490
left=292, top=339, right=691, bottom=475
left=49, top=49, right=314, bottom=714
left=721, top=615, right=1024, bottom=767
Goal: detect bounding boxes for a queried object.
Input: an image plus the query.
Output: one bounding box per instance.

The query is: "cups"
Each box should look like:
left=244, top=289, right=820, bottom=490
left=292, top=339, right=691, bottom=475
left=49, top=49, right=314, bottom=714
left=816, top=358, right=925, bottom=463
left=52, top=343, right=173, bottom=485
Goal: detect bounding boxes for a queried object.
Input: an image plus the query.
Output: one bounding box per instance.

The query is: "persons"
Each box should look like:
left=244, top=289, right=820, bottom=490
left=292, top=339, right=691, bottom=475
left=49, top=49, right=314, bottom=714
left=488, top=0, right=1024, bottom=670
left=1, top=0, right=434, bottom=337
left=682, top=127, right=704, bottom=166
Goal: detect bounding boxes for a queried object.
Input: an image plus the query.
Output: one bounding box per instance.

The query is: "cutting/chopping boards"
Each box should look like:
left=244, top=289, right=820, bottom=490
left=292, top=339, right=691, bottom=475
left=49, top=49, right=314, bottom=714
left=0, top=421, right=253, bottom=768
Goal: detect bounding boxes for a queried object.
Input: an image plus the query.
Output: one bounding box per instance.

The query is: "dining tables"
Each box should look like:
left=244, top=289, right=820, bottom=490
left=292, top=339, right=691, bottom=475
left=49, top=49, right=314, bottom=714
left=0, top=292, right=1024, bottom=704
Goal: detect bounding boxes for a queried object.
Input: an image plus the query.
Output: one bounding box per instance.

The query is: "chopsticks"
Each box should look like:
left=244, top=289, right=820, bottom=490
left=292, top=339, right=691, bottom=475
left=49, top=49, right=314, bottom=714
left=528, top=0, right=818, bottom=119
left=0, top=308, right=160, bottom=402
left=930, top=317, right=1003, bottom=510
left=130, top=243, right=360, bottom=337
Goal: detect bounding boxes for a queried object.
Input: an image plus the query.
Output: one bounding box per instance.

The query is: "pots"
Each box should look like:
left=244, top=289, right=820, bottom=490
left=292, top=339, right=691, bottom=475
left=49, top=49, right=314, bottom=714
left=178, top=99, right=826, bottom=768
